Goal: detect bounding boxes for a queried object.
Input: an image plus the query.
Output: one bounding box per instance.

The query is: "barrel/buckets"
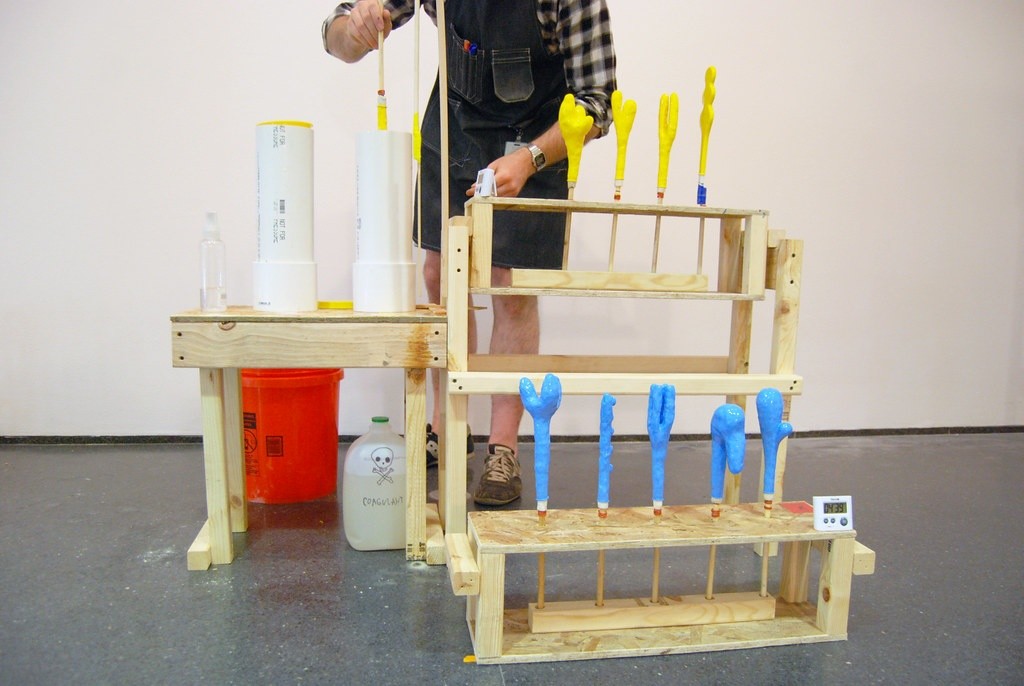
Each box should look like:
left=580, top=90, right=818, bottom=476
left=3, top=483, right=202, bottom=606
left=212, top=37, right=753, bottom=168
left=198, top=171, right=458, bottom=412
left=240, top=367, right=343, bottom=504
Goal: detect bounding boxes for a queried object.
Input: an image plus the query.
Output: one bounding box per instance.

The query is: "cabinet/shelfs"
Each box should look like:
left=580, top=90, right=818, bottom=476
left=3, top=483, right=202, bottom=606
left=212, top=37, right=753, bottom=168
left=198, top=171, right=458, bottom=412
left=427, top=195, right=875, bottom=664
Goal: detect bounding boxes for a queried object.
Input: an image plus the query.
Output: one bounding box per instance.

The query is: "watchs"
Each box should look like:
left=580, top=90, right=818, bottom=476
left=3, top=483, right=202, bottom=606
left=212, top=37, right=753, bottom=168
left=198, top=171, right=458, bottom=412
left=526, top=142, right=547, bottom=172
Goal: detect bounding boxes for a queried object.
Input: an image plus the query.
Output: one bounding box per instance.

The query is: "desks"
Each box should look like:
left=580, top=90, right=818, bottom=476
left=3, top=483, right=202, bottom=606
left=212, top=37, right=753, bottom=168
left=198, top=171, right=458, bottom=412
left=171, top=303, right=449, bottom=571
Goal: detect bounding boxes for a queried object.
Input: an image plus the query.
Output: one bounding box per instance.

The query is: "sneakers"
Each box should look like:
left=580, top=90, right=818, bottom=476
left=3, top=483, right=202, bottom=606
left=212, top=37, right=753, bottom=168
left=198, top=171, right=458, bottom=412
left=474, top=443, right=522, bottom=505
left=426, top=423, right=474, bottom=467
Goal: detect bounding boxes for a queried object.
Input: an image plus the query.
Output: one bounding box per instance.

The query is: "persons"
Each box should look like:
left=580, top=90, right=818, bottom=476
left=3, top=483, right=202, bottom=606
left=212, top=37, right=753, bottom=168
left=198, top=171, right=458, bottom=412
left=321, top=0, right=617, bottom=504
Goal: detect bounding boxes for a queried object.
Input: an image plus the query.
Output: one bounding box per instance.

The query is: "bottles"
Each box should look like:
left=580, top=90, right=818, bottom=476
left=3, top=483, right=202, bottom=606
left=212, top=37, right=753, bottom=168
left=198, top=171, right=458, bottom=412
left=342, top=418, right=408, bottom=551
left=199, top=212, right=227, bottom=312
